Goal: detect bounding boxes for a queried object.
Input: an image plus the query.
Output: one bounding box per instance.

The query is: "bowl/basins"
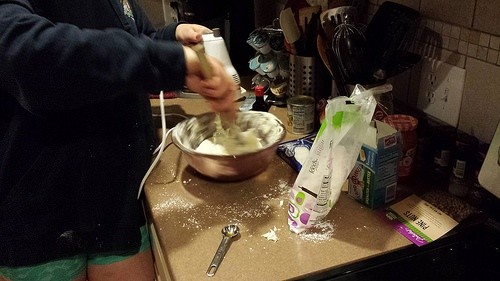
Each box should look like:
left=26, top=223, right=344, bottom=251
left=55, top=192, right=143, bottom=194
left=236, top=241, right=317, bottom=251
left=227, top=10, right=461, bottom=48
left=171, top=110, right=286, bottom=182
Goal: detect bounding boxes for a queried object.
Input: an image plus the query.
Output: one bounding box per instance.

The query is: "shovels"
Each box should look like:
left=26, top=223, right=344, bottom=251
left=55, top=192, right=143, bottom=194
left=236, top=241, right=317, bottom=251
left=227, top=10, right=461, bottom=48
left=320, top=5, right=353, bottom=48
left=298, top=4, right=321, bottom=34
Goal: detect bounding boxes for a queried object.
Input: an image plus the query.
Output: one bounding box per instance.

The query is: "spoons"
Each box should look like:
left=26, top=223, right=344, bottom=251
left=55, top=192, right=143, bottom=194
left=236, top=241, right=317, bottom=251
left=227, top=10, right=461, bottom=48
left=206, top=225, right=240, bottom=277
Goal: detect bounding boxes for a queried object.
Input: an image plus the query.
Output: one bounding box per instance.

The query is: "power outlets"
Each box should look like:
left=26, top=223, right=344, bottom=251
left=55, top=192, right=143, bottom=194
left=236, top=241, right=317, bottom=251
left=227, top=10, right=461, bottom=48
left=414, top=55, right=468, bottom=131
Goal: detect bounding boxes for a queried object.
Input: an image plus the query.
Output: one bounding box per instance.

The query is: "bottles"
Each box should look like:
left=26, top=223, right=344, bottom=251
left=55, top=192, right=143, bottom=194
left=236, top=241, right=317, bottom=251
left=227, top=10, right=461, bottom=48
left=253, top=86, right=269, bottom=112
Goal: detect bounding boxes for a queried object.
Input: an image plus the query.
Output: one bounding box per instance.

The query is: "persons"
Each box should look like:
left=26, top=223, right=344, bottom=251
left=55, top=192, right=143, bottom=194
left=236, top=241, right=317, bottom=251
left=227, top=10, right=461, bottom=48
left=0, top=0, right=242, bottom=281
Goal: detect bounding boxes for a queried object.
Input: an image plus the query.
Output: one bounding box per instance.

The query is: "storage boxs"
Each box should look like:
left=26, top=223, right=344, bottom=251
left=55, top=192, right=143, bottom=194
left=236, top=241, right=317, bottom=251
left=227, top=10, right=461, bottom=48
left=348, top=120, right=402, bottom=210
